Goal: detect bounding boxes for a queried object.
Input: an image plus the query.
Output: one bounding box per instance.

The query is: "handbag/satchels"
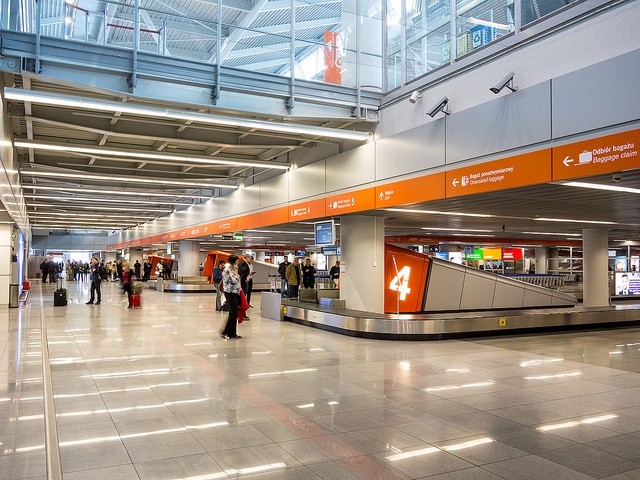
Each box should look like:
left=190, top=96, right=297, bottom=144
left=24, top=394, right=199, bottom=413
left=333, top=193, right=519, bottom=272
left=220, top=301, right=229, bottom=311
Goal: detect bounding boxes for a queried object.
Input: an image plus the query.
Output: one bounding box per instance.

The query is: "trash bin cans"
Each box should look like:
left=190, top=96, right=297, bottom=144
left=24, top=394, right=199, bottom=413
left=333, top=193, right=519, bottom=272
left=9, top=283, right=19, bottom=308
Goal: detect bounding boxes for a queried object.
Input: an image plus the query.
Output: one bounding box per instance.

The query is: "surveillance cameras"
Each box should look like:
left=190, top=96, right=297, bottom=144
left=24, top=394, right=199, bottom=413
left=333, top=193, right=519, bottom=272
left=426, top=96, right=449, bottom=118
left=612, top=173, right=623, bottom=182
left=409, top=90, right=425, bottom=103
left=490, top=71, right=515, bottom=94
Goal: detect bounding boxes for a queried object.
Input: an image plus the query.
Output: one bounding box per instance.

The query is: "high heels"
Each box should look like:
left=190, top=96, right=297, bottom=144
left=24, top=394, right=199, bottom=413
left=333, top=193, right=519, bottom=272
left=127, top=305, right=132, bottom=308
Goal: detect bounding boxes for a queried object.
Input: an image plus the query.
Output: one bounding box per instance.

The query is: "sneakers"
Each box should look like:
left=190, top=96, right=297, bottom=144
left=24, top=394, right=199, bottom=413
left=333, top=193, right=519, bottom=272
left=234, top=335, right=242, bottom=338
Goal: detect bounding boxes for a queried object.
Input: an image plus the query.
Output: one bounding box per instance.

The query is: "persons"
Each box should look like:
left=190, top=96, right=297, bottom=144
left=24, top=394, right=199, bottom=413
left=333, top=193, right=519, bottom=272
left=86, top=257, right=102, bottom=305
left=157, top=260, right=171, bottom=280
left=99, top=259, right=123, bottom=283
left=66, top=259, right=89, bottom=281
left=237, top=254, right=250, bottom=321
left=302, top=258, right=316, bottom=288
left=142, top=261, right=152, bottom=281
left=212, top=260, right=225, bottom=310
left=247, top=256, right=256, bottom=308
left=278, top=256, right=291, bottom=298
left=133, top=259, right=142, bottom=278
left=40, top=258, right=60, bottom=283
left=222, top=255, right=242, bottom=340
left=619, top=275, right=634, bottom=295
left=285, top=257, right=302, bottom=297
left=328, top=260, right=340, bottom=279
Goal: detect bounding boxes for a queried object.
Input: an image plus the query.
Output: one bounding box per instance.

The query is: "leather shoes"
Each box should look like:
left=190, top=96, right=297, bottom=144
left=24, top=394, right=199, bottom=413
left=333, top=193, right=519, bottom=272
left=86, top=301, right=93, bottom=304
left=95, top=301, right=100, bottom=305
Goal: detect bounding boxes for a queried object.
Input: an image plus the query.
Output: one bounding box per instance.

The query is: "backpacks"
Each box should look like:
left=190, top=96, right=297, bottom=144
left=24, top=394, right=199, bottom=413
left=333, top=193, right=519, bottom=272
left=99, top=266, right=108, bottom=280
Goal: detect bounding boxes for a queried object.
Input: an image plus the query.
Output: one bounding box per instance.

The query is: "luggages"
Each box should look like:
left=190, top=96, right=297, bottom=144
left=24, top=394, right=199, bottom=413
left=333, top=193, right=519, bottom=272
left=131, top=294, right=140, bottom=307
left=54, top=277, right=66, bottom=306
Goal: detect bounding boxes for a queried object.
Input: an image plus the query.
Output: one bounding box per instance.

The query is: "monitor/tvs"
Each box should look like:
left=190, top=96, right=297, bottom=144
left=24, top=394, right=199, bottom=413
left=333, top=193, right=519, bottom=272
left=314, top=220, right=336, bottom=246
